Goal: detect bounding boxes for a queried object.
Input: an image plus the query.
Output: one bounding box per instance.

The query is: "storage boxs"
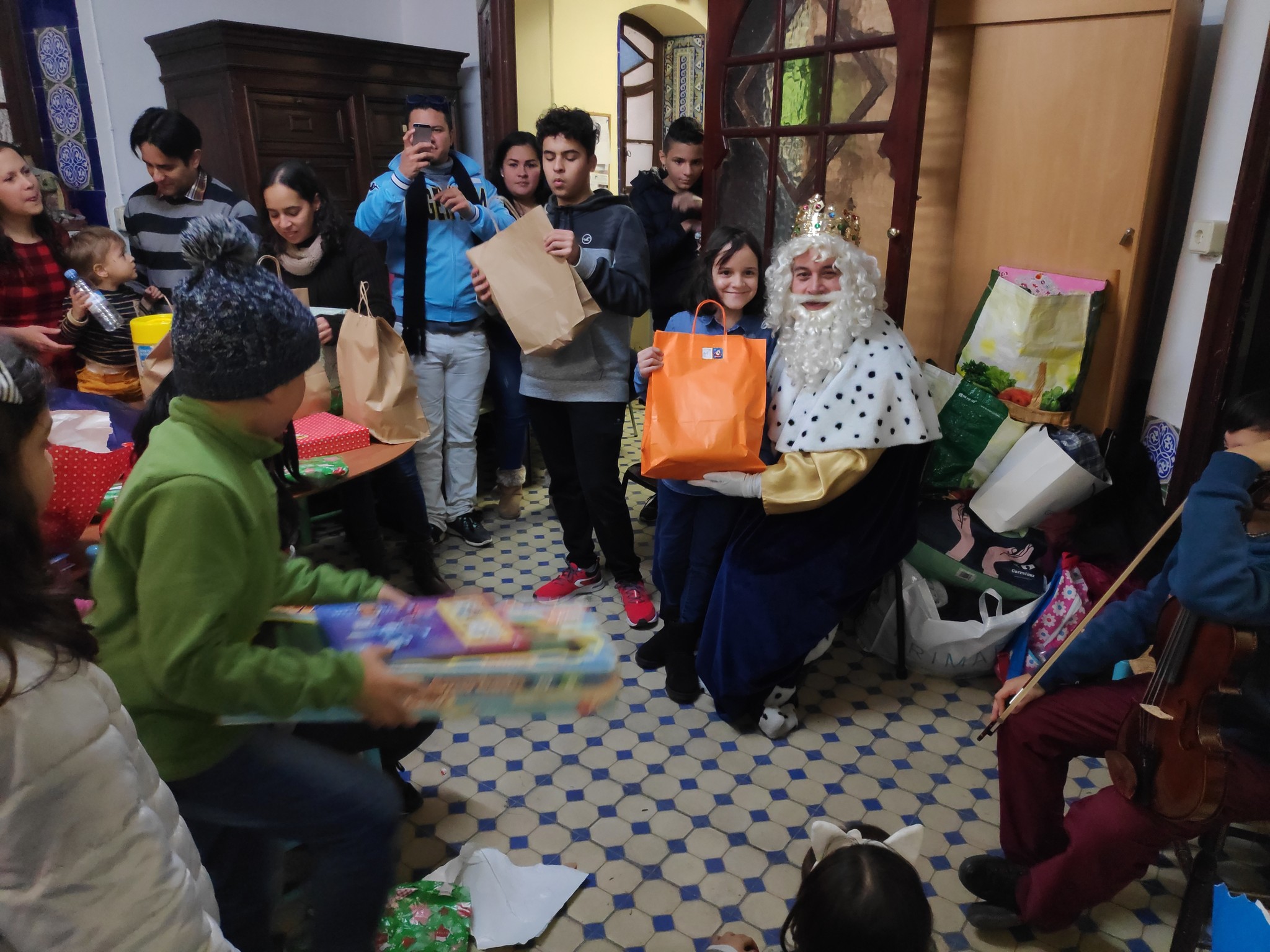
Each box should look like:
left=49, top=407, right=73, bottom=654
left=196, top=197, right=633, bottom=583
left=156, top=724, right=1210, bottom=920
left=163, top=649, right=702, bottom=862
left=293, top=411, right=370, bottom=458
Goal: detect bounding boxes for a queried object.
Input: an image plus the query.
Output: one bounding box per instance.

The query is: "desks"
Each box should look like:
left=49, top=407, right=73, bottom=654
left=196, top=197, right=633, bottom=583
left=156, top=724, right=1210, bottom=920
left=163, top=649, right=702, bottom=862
left=77, top=399, right=417, bottom=600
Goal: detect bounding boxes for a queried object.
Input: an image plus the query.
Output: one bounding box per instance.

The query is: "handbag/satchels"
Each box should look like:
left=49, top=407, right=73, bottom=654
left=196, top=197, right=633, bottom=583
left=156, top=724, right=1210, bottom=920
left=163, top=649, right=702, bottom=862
left=640, top=299, right=767, bottom=481
left=257, top=256, right=331, bottom=422
left=463, top=194, right=602, bottom=356
left=852, top=264, right=1165, bottom=683
left=335, top=280, right=431, bottom=444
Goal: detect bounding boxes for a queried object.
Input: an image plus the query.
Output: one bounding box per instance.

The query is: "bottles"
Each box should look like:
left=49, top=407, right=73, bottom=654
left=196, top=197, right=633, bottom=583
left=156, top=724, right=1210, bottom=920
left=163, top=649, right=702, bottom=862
left=64, top=268, right=125, bottom=332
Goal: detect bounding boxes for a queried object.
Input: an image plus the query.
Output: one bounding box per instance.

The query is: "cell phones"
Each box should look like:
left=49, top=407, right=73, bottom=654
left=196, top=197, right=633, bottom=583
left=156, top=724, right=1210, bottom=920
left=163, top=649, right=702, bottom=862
left=413, top=123, right=432, bottom=161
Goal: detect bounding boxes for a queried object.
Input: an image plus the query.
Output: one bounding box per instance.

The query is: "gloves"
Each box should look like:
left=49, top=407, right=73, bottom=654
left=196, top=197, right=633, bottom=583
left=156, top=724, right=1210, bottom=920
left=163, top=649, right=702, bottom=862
left=687, top=470, right=762, bottom=499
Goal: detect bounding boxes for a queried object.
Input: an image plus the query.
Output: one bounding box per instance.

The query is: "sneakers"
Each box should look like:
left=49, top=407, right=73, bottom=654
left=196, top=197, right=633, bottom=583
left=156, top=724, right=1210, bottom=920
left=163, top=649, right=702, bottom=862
left=445, top=514, right=492, bottom=547
left=430, top=524, right=446, bottom=545
left=614, top=578, right=658, bottom=628
left=531, top=555, right=605, bottom=603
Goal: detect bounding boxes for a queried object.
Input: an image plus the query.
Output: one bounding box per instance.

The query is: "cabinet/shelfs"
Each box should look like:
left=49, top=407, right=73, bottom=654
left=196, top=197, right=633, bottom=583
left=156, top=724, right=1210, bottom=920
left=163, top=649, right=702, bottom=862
left=144, top=19, right=470, bottom=226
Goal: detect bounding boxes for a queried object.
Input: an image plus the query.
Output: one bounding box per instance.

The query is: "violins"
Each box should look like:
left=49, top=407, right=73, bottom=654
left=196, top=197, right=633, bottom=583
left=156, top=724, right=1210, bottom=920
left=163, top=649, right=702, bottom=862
left=1103, top=496, right=1254, bottom=824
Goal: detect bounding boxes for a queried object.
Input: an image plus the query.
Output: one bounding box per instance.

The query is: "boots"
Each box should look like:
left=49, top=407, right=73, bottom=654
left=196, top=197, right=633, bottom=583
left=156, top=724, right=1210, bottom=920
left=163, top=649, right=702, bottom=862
left=496, top=465, right=526, bottom=520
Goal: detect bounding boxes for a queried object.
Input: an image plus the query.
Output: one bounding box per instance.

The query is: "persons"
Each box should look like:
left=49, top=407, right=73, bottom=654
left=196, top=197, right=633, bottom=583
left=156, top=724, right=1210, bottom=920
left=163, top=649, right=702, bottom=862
left=706, top=818, right=935, bottom=952
left=85, top=212, right=420, bottom=952
left=0, top=91, right=724, bottom=631
left=690, top=192, right=942, bottom=737
left=0, top=331, right=236, bottom=952
left=957, top=391, right=1270, bottom=936
left=633, top=225, right=775, bottom=707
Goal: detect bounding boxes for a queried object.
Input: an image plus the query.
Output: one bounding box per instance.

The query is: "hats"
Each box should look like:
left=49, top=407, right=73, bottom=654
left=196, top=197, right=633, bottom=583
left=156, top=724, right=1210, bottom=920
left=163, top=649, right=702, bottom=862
left=171, top=212, right=321, bottom=402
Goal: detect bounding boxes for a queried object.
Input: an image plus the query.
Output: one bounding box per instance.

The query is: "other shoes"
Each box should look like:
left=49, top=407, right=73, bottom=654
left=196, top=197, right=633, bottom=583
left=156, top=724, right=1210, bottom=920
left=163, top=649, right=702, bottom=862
left=636, top=623, right=668, bottom=671
left=663, top=637, right=700, bottom=703
left=955, top=854, right=1024, bottom=930
